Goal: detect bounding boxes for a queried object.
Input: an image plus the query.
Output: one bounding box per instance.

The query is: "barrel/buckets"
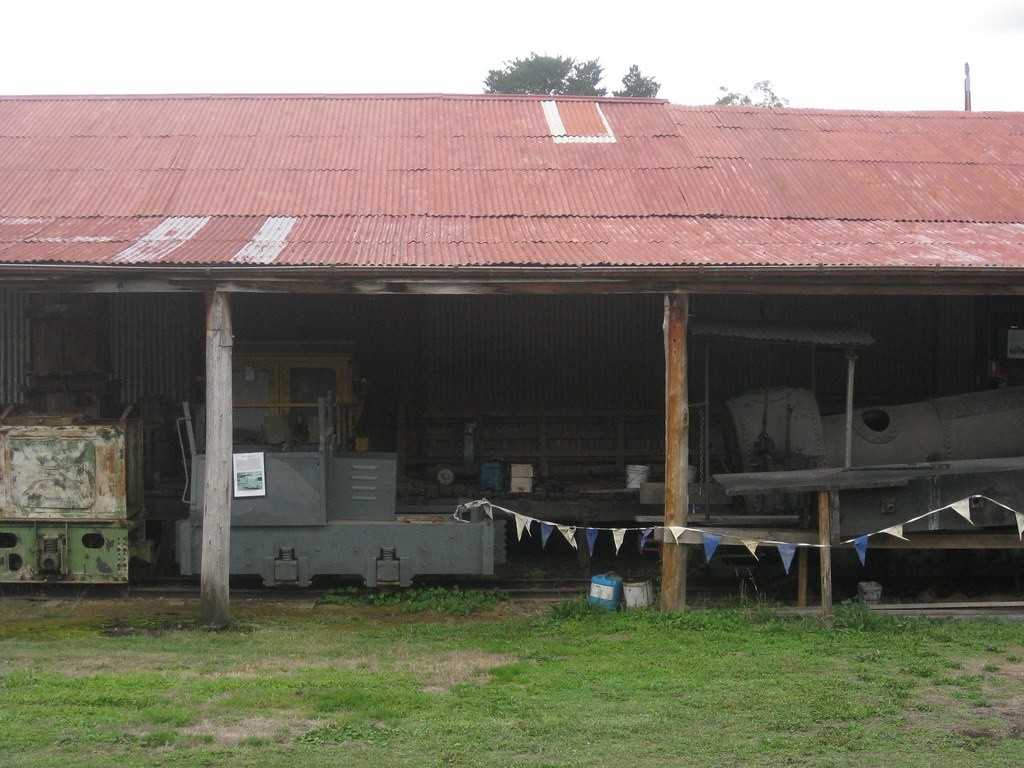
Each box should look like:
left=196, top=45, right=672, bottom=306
left=621, top=575, right=653, bottom=608
left=858, top=581, right=882, bottom=606
left=688, top=464, right=697, bottom=483
left=625, top=464, right=649, bottom=488
left=588, top=571, right=624, bottom=609
